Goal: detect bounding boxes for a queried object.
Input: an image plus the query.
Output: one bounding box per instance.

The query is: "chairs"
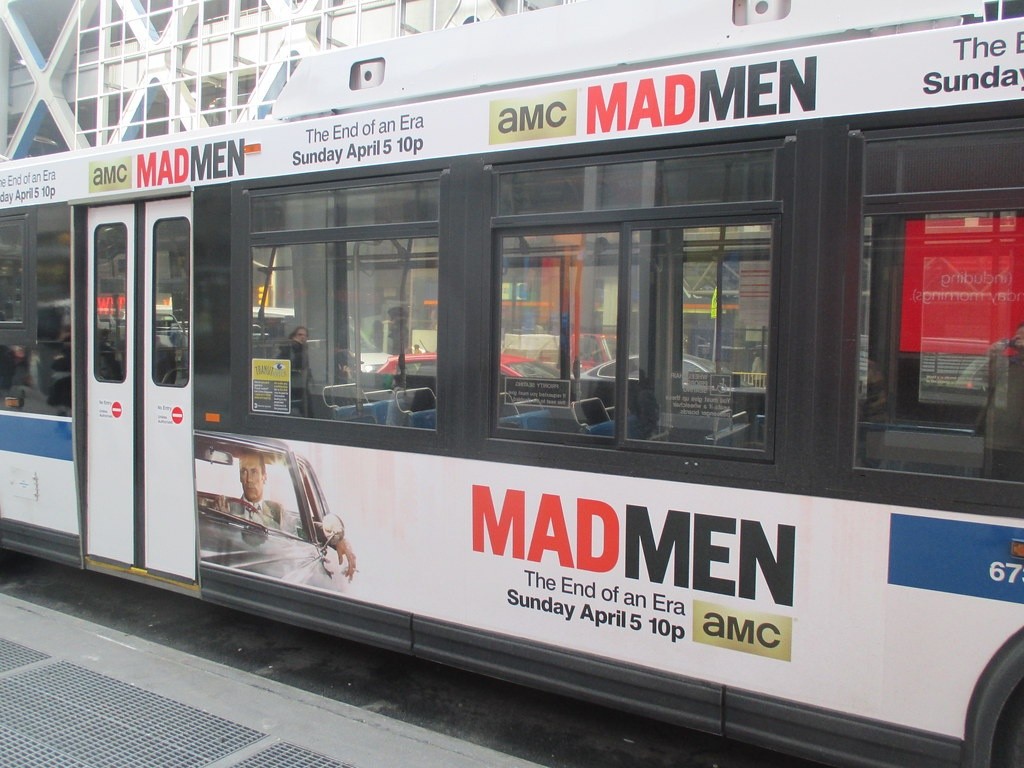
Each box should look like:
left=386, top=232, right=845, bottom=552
left=319, top=382, right=640, bottom=441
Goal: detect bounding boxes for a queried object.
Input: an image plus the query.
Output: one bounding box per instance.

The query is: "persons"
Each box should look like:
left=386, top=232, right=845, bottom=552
left=275, top=326, right=314, bottom=419
left=333, top=349, right=365, bottom=386
left=210, top=452, right=357, bottom=582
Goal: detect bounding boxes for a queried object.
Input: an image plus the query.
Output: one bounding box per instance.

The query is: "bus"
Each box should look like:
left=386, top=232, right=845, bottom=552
left=0, top=0, right=1024, bottom=768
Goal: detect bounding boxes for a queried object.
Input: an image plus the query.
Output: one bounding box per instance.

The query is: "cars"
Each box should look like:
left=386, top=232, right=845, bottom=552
left=570, top=352, right=750, bottom=389
left=376, top=354, right=560, bottom=378
